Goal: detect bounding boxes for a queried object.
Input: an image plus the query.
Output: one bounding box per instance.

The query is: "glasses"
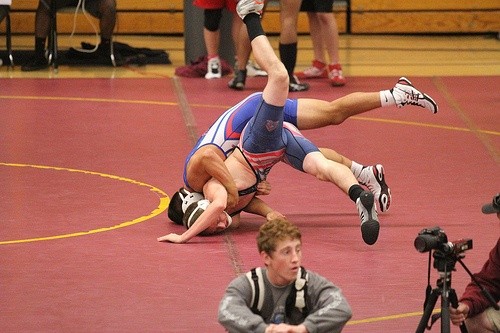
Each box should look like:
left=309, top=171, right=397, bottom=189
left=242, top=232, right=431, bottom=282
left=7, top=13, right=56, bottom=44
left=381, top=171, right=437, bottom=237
left=493, top=194, right=500, bottom=211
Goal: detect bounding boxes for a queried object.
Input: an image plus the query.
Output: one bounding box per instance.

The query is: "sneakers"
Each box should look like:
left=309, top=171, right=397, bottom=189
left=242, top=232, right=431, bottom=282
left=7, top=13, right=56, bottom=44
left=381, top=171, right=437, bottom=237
left=245, top=60, right=269, bottom=76
left=237, top=0, right=265, bottom=20
left=204, top=57, right=222, bottom=80
left=327, top=64, right=345, bottom=86
left=288, top=74, right=309, bottom=92
left=228, top=69, right=247, bottom=88
left=354, top=189, right=381, bottom=246
left=391, top=76, right=438, bottom=114
left=221, top=59, right=235, bottom=77
left=293, top=60, right=327, bottom=79
left=174, top=56, right=208, bottom=77
left=356, top=164, right=392, bottom=212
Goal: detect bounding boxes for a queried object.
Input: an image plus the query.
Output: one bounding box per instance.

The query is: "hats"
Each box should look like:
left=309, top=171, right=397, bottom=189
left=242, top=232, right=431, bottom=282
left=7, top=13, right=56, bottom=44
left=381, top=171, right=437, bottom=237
left=482, top=197, right=500, bottom=214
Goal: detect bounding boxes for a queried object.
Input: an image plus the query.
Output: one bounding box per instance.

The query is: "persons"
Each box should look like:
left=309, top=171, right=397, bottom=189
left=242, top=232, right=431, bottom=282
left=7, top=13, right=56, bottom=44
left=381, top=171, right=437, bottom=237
left=216, top=217, right=352, bottom=333
left=158, top=0, right=379, bottom=247
left=23, top=0, right=125, bottom=70
left=192, top=0, right=267, bottom=79
left=299, top=0, right=346, bottom=86
left=449, top=192, right=500, bottom=333
left=169, top=77, right=438, bottom=225
left=227, top=0, right=309, bottom=92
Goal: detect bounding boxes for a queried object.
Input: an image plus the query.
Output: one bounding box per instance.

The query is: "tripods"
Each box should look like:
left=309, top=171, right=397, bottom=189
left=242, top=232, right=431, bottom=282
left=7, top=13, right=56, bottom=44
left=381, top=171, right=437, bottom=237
left=414, top=273, right=469, bottom=333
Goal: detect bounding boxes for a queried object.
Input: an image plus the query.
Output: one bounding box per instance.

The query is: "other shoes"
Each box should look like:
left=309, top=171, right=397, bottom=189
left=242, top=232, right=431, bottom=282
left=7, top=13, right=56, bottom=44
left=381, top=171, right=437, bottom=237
left=96, top=42, right=125, bottom=65
left=21, top=49, right=49, bottom=71
